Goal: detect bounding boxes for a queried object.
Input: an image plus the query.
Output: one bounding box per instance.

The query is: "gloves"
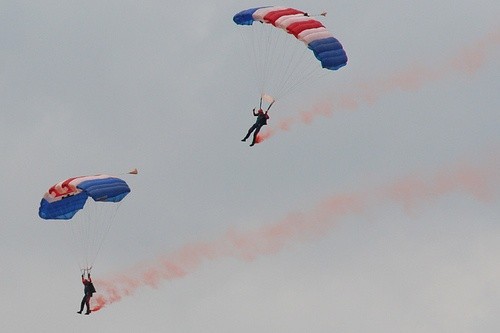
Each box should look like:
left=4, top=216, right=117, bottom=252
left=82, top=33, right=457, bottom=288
left=253, top=108, right=255, bottom=111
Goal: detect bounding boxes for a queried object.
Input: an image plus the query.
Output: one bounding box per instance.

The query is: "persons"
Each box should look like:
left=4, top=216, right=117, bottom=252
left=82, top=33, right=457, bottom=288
left=240, top=98, right=276, bottom=147
left=76, top=273, right=96, bottom=315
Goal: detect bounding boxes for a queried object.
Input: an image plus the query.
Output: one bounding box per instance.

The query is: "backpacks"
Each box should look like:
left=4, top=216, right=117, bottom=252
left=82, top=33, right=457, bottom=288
left=86, top=282, right=96, bottom=293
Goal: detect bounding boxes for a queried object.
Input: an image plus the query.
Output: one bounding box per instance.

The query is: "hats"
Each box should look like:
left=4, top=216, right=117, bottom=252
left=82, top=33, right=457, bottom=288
left=258, top=109, right=263, bottom=113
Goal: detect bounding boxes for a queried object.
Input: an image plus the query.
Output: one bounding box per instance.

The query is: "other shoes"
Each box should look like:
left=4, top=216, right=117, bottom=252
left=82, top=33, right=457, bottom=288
left=250, top=144, right=254, bottom=146
left=242, top=139, right=246, bottom=141
left=77, top=311, right=81, bottom=313
left=86, top=310, right=91, bottom=314
